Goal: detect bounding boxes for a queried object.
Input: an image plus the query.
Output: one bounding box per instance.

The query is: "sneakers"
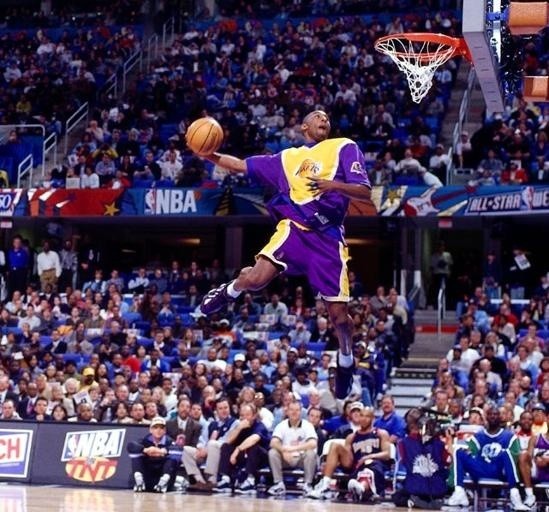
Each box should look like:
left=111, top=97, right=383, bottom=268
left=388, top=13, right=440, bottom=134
left=335, top=351, right=355, bottom=399
left=200, top=280, right=239, bottom=314
left=150, top=417, right=166, bottom=426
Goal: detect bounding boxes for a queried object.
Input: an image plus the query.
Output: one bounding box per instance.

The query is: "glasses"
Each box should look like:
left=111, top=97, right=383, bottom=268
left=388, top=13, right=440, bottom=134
left=153, top=424, right=165, bottom=429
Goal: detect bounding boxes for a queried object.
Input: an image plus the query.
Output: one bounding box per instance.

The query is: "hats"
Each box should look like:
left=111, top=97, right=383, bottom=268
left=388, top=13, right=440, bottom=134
left=234, top=353, right=245, bottom=362
left=351, top=402, right=364, bottom=411
left=532, top=403, right=545, bottom=410
left=83, top=368, right=95, bottom=375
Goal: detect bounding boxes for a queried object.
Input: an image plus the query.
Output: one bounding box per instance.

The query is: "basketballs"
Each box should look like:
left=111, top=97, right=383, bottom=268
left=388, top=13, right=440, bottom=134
left=184, top=116, right=225, bottom=156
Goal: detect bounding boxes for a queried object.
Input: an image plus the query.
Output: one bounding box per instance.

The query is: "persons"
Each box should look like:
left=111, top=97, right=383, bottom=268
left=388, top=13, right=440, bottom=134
left=1, top=223, right=548, bottom=512
left=199, top=108, right=371, bottom=398
left=0, top=1, right=549, bottom=190
